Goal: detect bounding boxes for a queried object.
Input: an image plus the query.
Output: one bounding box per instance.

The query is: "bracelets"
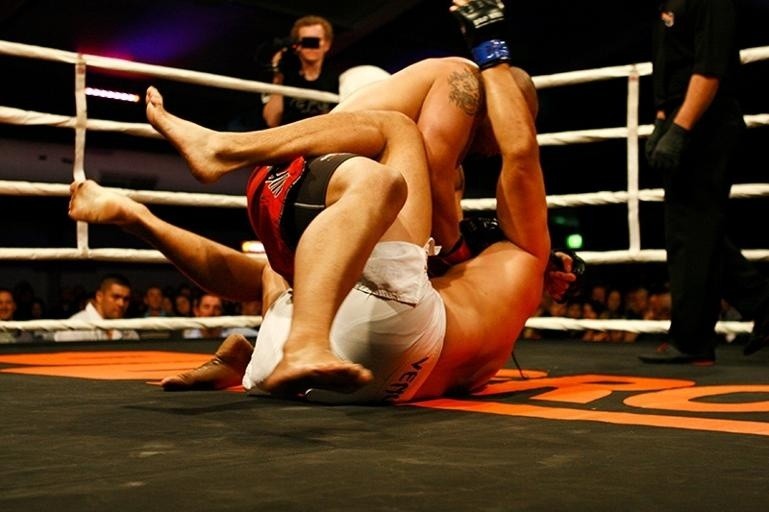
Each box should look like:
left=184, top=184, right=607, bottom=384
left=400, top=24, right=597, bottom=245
left=440, top=235, right=464, bottom=257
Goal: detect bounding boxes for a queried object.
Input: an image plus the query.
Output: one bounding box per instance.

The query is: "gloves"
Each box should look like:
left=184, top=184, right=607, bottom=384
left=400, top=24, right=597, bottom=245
left=644, top=119, right=688, bottom=173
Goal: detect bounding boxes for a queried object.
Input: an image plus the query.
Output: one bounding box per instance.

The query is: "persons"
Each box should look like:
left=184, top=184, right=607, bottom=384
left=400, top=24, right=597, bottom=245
left=262, top=16, right=338, bottom=127
left=158, top=57, right=578, bottom=396
left=0, top=269, right=262, bottom=346
left=517, top=273, right=748, bottom=344
left=69, top=1, right=551, bottom=403
left=639, top=0, right=768, bottom=367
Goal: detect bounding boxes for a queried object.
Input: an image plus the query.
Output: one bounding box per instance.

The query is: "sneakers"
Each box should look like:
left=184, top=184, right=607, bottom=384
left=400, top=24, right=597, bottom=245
left=745, top=312, right=769, bottom=353
left=638, top=343, right=715, bottom=366
